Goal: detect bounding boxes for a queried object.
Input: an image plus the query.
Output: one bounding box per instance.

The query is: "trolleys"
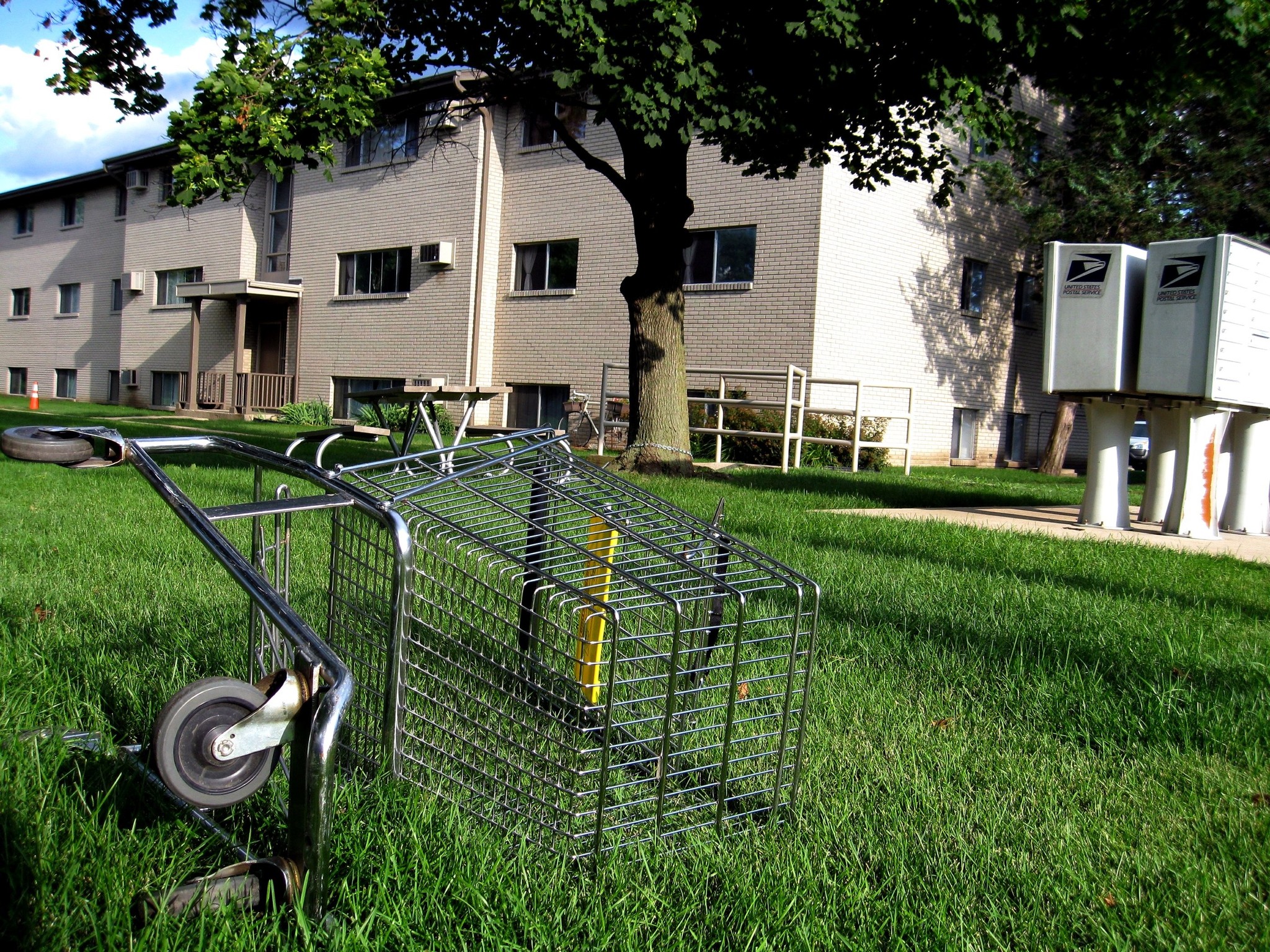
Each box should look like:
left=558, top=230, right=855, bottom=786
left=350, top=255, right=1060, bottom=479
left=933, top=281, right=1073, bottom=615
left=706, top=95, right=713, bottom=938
left=0, top=421, right=824, bottom=930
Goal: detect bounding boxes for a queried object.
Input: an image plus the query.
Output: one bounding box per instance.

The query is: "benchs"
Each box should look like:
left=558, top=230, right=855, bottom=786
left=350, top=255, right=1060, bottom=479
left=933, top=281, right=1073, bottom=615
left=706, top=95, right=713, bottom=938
left=287, top=425, right=399, bottom=479
left=452, top=424, right=575, bottom=484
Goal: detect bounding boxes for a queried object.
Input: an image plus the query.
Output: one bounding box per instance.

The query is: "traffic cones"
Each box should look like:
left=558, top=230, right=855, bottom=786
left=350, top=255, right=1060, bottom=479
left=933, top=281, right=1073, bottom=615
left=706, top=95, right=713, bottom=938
left=30, top=381, right=40, bottom=410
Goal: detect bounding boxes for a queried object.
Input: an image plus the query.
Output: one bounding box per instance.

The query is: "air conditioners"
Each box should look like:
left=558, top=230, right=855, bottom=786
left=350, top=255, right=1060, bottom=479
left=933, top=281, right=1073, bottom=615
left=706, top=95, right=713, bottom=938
left=419, top=241, right=452, bottom=265
left=127, top=171, right=148, bottom=190
left=412, top=378, right=445, bottom=405
left=121, top=368, right=141, bottom=386
left=121, top=272, right=144, bottom=290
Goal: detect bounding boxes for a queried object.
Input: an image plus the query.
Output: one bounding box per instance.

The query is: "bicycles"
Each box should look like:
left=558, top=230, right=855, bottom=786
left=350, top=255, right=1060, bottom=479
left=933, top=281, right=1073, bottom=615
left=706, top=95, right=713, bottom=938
left=557, top=390, right=629, bottom=450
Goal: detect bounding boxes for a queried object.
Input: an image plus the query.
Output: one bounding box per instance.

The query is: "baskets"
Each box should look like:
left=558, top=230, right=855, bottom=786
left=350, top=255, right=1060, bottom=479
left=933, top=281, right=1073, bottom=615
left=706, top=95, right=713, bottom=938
left=606, top=401, right=629, bottom=414
left=563, top=401, right=584, bottom=412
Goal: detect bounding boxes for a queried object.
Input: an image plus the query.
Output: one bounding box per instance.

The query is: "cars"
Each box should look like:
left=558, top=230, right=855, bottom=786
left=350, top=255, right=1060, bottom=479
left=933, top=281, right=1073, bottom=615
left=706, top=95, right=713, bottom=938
left=1127, top=421, right=1148, bottom=471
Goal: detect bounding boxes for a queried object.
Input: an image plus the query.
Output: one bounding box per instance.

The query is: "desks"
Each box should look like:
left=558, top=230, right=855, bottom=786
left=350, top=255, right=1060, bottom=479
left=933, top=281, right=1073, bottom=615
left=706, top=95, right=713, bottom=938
left=341, top=386, right=522, bottom=495
